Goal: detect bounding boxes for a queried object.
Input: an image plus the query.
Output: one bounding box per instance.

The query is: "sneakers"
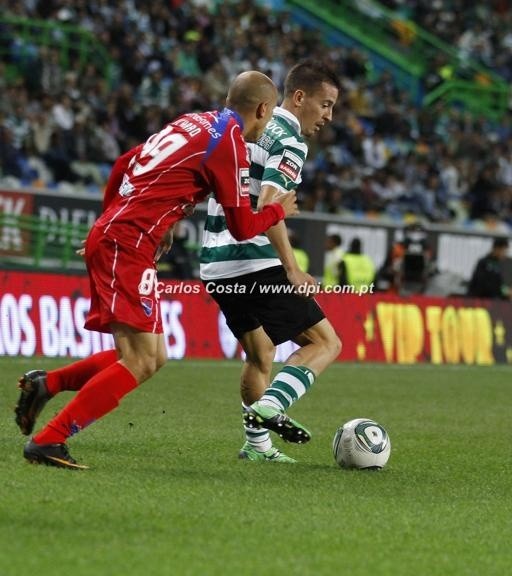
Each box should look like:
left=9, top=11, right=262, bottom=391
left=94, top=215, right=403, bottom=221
left=241, top=400, right=311, bottom=444
left=23, top=438, right=86, bottom=469
left=14, top=370, right=54, bottom=435
left=237, top=440, right=296, bottom=463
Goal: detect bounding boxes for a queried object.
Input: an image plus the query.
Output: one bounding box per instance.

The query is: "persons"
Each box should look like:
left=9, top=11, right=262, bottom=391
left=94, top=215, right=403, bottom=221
left=16, top=60, right=344, bottom=471
left=0, top=0, right=512, bottom=300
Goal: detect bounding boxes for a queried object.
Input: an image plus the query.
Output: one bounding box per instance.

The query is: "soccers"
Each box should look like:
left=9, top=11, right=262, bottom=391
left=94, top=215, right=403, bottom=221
left=333, top=418, right=391, bottom=471
